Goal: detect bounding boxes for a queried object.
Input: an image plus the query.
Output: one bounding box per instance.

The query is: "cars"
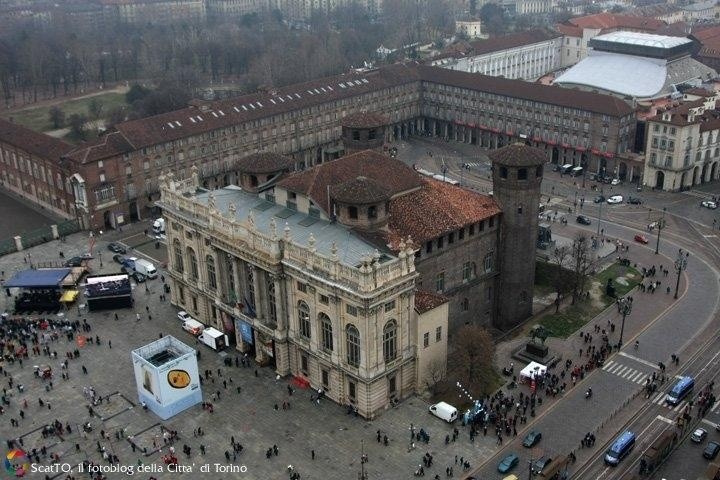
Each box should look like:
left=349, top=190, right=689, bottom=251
left=133, top=272, right=146, bottom=282
left=522, top=431, right=541, bottom=448
left=498, top=453, right=519, bottom=473
left=702, top=201, right=717, bottom=209
left=635, top=233, right=649, bottom=244
left=501, top=474, right=519, bottom=480
left=413, top=162, right=421, bottom=170
left=690, top=427, right=707, bottom=443
left=576, top=214, right=591, bottom=225
left=703, top=441, right=720, bottom=459
left=113, top=255, right=125, bottom=264
left=108, top=242, right=119, bottom=252
left=588, top=172, right=643, bottom=206
left=121, top=266, right=136, bottom=276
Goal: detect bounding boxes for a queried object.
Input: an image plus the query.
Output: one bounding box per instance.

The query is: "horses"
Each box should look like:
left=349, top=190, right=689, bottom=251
left=529, top=329, right=554, bottom=346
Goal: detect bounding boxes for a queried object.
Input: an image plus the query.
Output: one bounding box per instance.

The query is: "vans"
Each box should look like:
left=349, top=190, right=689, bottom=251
left=666, top=376, right=694, bottom=406
left=126, top=257, right=137, bottom=269
left=560, top=164, right=573, bottom=174
left=604, top=431, right=636, bottom=467
left=570, top=167, right=583, bottom=176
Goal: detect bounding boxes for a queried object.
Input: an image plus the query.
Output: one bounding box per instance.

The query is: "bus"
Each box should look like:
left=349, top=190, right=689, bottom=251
left=416, top=168, right=460, bottom=188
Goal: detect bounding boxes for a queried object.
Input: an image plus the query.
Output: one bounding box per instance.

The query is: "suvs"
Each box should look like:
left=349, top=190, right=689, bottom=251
left=177, top=311, right=191, bottom=321
left=531, top=455, right=552, bottom=476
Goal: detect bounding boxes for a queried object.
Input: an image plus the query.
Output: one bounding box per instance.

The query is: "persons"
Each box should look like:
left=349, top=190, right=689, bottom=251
left=388, top=130, right=719, bottom=480
left=0, top=227, right=389, bottom=480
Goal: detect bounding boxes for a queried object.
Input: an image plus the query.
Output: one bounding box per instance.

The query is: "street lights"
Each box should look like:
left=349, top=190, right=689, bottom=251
left=673, top=249, right=690, bottom=298
left=616, top=295, right=633, bottom=345
left=440, top=165, right=448, bottom=183
left=655, top=218, right=667, bottom=254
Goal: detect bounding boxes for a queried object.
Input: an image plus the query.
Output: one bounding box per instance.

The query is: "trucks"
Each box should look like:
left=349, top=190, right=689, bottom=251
left=152, top=218, right=166, bottom=234
left=198, top=326, right=230, bottom=352
left=135, top=258, right=158, bottom=279
left=181, top=319, right=205, bottom=336
left=428, top=401, right=458, bottom=423
